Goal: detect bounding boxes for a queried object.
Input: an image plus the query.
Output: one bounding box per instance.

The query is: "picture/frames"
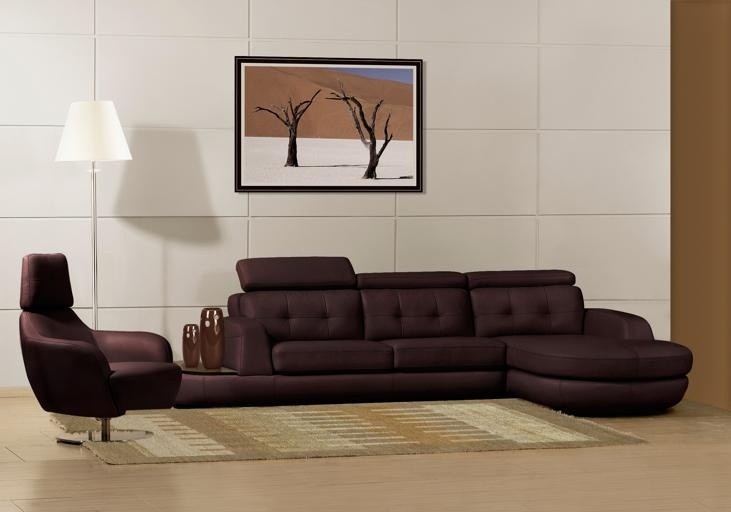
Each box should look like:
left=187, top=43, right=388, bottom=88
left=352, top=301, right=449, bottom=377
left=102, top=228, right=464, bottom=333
left=231, top=54, right=425, bottom=195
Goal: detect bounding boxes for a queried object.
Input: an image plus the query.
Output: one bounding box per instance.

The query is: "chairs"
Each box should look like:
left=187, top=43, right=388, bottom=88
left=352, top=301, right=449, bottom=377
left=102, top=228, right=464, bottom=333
left=19, top=251, right=181, bottom=446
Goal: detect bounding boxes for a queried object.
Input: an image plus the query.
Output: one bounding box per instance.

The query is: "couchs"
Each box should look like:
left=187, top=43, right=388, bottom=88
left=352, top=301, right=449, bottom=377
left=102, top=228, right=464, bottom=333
left=175, top=256, right=691, bottom=416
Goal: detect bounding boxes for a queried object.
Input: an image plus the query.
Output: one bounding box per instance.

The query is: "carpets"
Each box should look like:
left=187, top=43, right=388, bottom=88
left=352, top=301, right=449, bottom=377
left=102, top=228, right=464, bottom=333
left=44, top=397, right=649, bottom=467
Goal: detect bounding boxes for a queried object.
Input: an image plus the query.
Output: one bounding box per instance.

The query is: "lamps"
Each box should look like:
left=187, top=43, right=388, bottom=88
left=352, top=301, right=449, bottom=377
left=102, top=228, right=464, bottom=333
left=56, top=100, right=133, bottom=327
